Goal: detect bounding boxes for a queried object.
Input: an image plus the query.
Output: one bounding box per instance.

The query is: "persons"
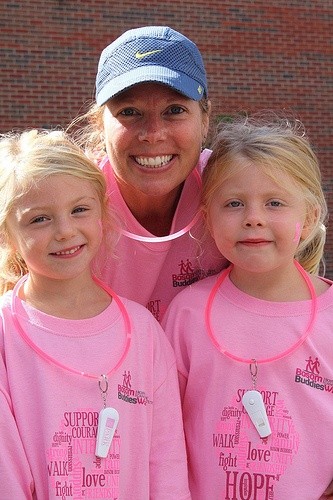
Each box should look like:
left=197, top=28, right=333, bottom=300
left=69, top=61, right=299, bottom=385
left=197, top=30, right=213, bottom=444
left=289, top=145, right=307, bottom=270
left=72, top=26, right=231, bottom=325
left=160, top=109, right=333, bottom=500
left=0, top=129, right=188, bottom=500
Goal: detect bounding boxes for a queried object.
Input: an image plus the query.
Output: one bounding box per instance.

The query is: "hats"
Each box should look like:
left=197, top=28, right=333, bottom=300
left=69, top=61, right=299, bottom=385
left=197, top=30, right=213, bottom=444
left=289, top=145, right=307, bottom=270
left=95, top=26, right=208, bottom=107
